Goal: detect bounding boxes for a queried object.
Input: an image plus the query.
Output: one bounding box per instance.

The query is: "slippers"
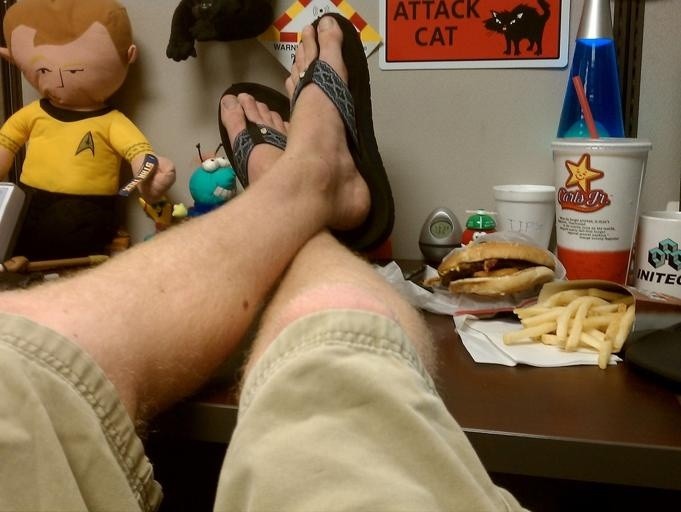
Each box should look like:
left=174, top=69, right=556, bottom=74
left=217, top=80, right=292, bottom=192
left=289, top=11, right=396, bottom=252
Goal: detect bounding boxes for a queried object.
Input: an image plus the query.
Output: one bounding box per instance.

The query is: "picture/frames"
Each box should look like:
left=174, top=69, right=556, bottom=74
left=376, top=0, right=570, bottom=71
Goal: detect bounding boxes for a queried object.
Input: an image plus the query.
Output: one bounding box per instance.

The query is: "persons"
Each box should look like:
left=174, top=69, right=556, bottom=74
left=1, top=1, right=176, bottom=262
left=0, top=14, right=529, bottom=512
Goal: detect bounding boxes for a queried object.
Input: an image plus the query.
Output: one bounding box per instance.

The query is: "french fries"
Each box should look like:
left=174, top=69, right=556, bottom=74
left=502, top=287, right=635, bottom=371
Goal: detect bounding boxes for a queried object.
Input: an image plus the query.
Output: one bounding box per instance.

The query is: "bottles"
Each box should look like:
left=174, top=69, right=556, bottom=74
left=556, top=0, right=627, bottom=138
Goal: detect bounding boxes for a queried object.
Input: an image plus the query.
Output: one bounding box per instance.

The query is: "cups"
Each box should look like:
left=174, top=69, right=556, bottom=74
left=551, top=137, right=654, bottom=287
left=492, top=183, right=561, bottom=250
left=637, top=200, right=681, bottom=305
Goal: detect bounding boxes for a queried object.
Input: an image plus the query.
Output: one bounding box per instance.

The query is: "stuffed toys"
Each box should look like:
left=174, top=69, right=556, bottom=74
left=188, top=141, right=237, bottom=219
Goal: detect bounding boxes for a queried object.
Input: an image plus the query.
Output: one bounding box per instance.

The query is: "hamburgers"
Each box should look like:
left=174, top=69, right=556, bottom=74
left=437, top=240, right=556, bottom=297
